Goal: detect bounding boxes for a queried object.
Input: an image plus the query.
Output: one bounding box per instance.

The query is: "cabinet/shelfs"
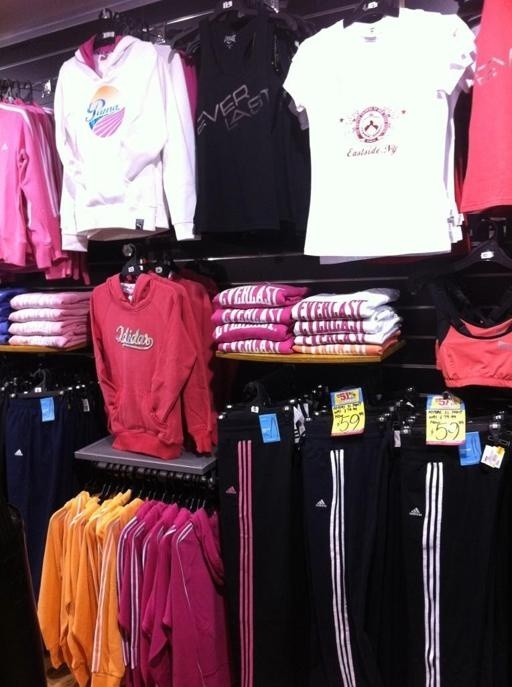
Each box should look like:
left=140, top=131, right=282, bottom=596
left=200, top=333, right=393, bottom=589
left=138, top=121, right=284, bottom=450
left=0, top=0, right=511, bottom=474
